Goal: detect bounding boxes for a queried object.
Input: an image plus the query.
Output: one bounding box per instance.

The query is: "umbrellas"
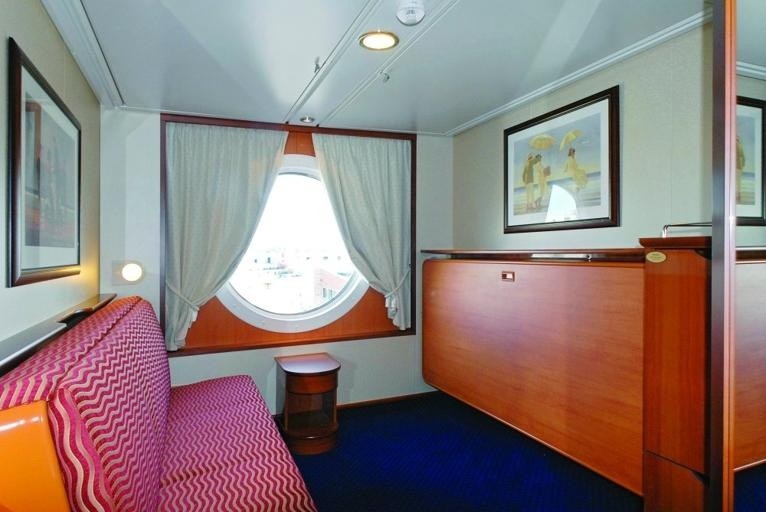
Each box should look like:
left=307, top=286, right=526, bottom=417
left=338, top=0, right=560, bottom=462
left=529, top=134, right=557, bottom=150
left=560, top=129, right=584, bottom=159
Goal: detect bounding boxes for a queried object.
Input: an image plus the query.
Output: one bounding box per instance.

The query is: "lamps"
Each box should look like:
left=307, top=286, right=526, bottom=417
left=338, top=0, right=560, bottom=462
left=395, top=1, right=426, bottom=25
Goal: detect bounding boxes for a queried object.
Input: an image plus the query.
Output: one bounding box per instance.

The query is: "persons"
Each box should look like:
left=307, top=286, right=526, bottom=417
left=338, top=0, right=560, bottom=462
left=522, top=152, right=551, bottom=212
left=564, top=148, right=580, bottom=191
left=735, top=134, right=745, bottom=200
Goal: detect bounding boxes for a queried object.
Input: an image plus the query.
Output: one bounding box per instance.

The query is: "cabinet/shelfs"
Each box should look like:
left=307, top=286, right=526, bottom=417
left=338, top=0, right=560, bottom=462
left=273, top=352, right=341, bottom=456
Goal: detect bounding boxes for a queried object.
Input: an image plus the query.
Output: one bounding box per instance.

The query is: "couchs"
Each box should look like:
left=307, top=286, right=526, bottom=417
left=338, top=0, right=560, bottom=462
left=0, top=289, right=315, bottom=512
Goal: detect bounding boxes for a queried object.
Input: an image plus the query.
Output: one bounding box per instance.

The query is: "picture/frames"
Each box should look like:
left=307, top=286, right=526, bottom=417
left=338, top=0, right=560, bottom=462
left=734, top=94, right=766, bottom=227
left=4, top=35, right=82, bottom=286
left=502, top=84, right=620, bottom=235
